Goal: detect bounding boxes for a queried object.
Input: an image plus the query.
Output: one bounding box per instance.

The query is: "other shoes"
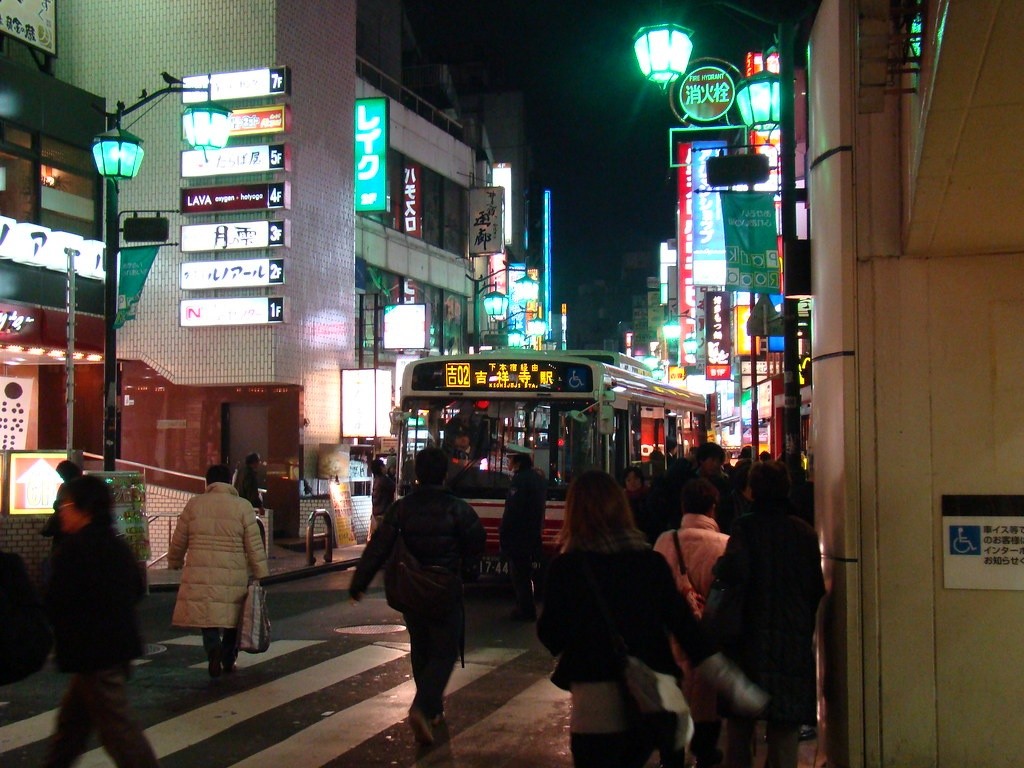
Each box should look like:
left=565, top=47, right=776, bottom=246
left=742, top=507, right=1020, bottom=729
left=798, top=729, right=816, bottom=740
left=695, top=748, right=724, bottom=768
left=509, top=607, right=537, bottom=622
left=207, top=647, right=222, bottom=676
left=408, top=707, right=435, bottom=744
left=431, top=712, right=446, bottom=726
left=222, top=661, right=237, bottom=673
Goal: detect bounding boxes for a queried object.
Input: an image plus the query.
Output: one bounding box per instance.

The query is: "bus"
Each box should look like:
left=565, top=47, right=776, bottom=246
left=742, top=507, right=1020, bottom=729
left=390, top=345, right=722, bottom=596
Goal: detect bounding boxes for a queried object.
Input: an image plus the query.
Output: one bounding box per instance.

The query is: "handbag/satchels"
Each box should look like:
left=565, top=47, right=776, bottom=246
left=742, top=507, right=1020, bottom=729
left=679, top=574, right=706, bottom=621
left=238, top=580, right=272, bottom=653
left=618, top=655, right=696, bottom=752
left=386, top=537, right=464, bottom=614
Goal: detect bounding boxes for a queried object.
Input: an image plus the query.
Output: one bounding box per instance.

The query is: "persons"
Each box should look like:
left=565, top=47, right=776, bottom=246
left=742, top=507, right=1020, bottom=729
left=235, top=453, right=264, bottom=515
left=439, top=400, right=493, bottom=496
left=0, top=460, right=162, bottom=768
left=499, top=454, right=547, bottom=622
left=537, top=469, right=745, bottom=768
left=712, top=446, right=829, bottom=768
left=622, top=442, right=753, bottom=541
left=167, top=464, right=273, bottom=679
left=349, top=448, right=488, bottom=745
left=371, top=457, right=396, bottom=534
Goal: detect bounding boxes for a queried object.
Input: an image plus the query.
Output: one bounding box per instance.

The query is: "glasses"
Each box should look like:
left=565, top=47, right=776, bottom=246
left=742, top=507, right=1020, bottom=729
left=55, top=500, right=75, bottom=512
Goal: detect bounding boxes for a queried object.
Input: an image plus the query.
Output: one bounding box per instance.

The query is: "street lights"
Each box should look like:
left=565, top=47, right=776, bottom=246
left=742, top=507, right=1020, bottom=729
left=466, top=267, right=540, bottom=353
left=91, top=86, right=204, bottom=467
